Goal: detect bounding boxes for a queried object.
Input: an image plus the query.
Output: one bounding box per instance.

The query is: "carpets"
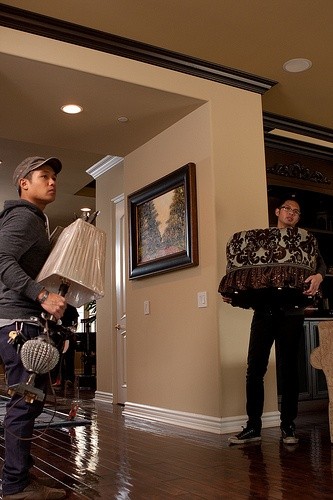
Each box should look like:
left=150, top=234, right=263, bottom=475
left=0, top=390, right=92, bottom=434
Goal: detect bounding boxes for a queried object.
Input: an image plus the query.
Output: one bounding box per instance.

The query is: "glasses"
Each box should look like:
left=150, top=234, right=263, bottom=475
left=280, top=206, right=301, bottom=216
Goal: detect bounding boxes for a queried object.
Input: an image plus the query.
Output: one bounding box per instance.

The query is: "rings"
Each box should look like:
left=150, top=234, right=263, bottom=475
left=314, top=289, right=319, bottom=292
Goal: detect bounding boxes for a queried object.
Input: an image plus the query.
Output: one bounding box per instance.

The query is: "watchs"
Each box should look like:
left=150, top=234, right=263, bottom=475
left=39, top=289, right=50, bottom=304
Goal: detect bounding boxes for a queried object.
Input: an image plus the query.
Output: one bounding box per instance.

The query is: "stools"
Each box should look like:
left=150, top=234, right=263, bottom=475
left=217, top=227, right=324, bottom=311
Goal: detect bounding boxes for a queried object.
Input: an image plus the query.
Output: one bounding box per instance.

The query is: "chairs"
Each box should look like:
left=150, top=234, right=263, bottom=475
left=310, top=321, right=333, bottom=475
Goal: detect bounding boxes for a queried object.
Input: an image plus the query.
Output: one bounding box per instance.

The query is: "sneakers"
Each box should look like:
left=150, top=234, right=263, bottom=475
left=281, top=425, right=299, bottom=443
left=227, top=426, right=262, bottom=443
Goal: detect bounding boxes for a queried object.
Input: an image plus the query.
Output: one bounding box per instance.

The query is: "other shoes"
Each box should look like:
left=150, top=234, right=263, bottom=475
left=26, top=472, right=58, bottom=487
left=2, top=480, right=66, bottom=500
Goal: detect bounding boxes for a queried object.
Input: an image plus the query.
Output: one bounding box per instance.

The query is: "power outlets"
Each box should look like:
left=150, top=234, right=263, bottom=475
left=197, top=291, right=207, bottom=309
left=143, top=300, right=150, bottom=315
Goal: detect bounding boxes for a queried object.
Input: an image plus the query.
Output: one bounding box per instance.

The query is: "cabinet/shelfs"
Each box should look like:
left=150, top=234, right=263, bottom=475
left=266, top=173, right=333, bottom=316
left=275, top=317, right=333, bottom=403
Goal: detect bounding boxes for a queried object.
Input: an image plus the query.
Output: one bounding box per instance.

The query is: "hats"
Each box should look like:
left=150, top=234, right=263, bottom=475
left=13, top=156, right=62, bottom=193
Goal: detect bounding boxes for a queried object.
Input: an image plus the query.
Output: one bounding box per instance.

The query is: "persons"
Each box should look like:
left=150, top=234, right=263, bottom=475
left=223, top=197, right=326, bottom=444
left=0, top=157, right=68, bottom=500
left=46, top=298, right=79, bottom=387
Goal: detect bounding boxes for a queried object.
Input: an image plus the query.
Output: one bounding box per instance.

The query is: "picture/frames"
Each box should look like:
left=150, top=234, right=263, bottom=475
left=127, top=162, right=199, bottom=281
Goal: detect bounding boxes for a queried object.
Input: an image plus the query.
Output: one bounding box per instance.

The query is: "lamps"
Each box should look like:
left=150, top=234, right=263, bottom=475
left=7, top=209, right=107, bottom=407
